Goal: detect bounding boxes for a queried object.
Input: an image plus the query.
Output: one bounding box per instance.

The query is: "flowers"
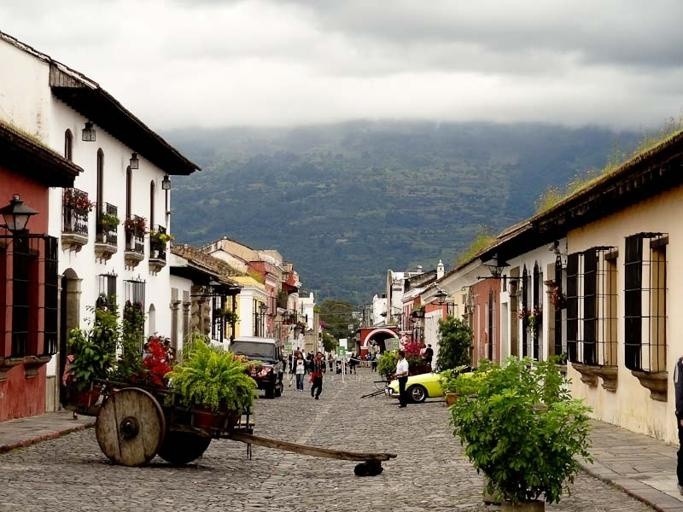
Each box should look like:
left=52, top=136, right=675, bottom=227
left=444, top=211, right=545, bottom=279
left=548, top=286, right=566, bottom=309
left=61, top=188, right=97, bottom=224
left=124, top=213, right=147, bottom=244
left=511, top=304, right=541, bottom=339
left=98, top=211, right=119, bottom=235
left=149, top=225, right=175, bottom=252
left=403, top=340, right=428, bottom=369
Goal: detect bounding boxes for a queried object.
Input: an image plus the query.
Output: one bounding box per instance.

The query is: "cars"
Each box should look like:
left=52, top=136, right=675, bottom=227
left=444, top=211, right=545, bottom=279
left=382, top=363, right=476, bottom=406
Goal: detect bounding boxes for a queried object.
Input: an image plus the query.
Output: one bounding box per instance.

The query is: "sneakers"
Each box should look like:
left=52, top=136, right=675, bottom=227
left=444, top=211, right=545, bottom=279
left=398, top=404, right=406, bottom=408
left=311, top=390, right=319, bottom=400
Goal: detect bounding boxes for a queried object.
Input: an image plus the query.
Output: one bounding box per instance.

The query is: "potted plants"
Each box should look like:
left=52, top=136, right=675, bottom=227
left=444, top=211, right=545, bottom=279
left=62, top=300, right=264, bottom=431
left=436, top=352, right=598, bottom=512
left=375, top=348, right=404, bottom=385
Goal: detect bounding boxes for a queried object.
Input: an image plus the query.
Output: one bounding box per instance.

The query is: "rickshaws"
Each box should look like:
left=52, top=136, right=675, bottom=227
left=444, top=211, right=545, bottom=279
left=58, top=373, right=399, bottom=468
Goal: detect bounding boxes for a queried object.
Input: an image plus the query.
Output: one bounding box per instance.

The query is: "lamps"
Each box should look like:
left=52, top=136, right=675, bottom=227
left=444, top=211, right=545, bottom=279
left=128, top=150, right=139, bottom=169
left=161, top=172, right=171, bottom=190
left=430, top=291, right=457, bottom=306
left=414, top=306, right=431, bottom=318
left=81, top=117, right=95, bottom=141
left=474, top=252, right=530, bottom=281
left=0, top=193, right=47, bottom=240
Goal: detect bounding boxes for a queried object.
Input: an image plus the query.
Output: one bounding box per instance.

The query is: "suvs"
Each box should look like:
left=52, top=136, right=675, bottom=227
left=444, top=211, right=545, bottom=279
left=227, top=335, right=286, bottom=399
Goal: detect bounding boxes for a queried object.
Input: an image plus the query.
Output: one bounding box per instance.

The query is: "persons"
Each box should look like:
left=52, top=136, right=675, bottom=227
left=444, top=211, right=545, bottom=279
left=671, top=357, right=683, bottom=497
left=307, top=351, right=327, bottom=401
left=419, top=344, right=427, bottom=360
left=389, top=350, right=409, bottom=409
left=284, top=347, right=357, bottom=392
left=370, top=349, right=380, bottom=373
left=423, top=343, right=433, bottom=367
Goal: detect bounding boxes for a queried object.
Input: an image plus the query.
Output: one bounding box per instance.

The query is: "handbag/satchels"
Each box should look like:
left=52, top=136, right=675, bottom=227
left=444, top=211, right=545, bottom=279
left=308, top=377, right=312, bottom=383
left=289, top=379, right=293, bottom=387
